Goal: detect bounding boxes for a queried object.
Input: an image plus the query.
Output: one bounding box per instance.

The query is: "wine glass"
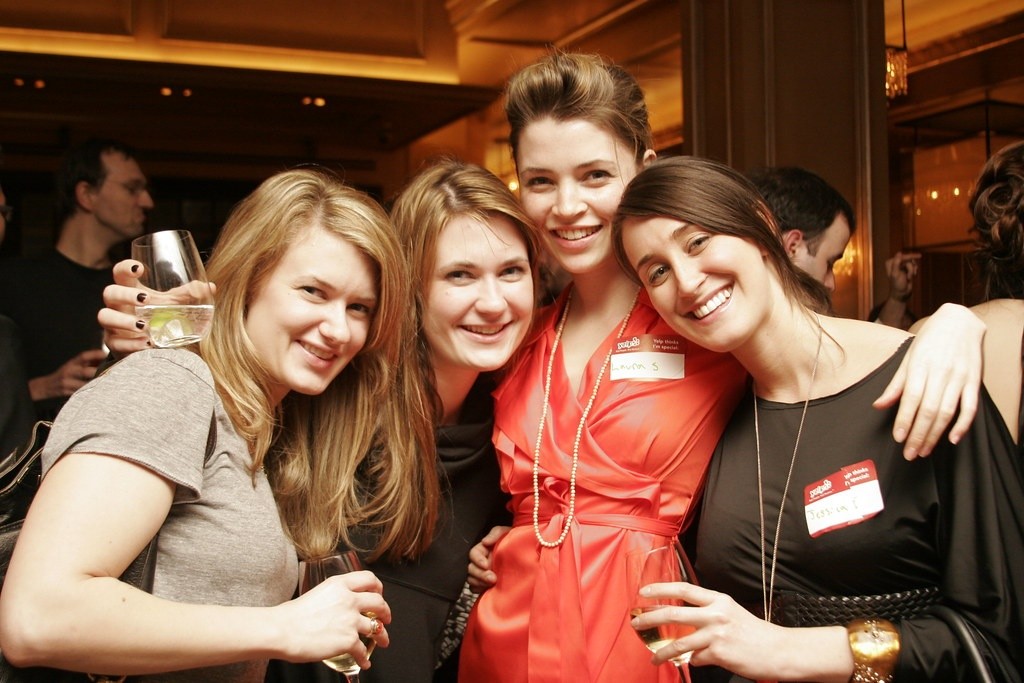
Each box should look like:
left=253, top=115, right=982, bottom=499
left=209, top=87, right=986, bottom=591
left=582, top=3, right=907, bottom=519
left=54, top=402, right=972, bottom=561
left=626, top=543, right=697, bottom=683
left=299, top=550, right=381, bottom=683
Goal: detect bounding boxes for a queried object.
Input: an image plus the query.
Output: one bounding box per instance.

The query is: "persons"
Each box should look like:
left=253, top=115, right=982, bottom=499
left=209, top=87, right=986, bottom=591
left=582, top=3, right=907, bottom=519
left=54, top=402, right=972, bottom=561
left=1, top=45, right=1024, bottom=683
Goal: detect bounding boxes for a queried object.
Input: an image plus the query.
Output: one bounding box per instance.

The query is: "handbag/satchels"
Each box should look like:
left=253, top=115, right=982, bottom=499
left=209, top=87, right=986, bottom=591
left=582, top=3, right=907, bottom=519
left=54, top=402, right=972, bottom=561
left=0, top=348, right=217, bottom=683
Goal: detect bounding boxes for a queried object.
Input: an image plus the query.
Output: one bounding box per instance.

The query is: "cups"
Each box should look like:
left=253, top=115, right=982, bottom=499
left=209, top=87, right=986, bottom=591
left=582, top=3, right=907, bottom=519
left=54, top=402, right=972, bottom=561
left=131, top=230, right=214, bottom=347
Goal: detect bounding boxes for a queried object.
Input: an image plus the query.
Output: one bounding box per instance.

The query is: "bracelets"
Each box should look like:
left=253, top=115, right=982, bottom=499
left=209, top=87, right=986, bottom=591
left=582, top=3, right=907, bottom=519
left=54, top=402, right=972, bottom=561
left=843, top=616, right=900, bottom=683
left=891, top=289, right=912, bottom=302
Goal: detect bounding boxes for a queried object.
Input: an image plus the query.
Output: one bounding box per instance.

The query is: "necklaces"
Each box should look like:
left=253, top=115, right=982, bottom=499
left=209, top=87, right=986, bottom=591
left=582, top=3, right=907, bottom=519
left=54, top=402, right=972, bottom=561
left=753, top=308, right=824, bottom=624
left=532, top=279, right=641, bottom=547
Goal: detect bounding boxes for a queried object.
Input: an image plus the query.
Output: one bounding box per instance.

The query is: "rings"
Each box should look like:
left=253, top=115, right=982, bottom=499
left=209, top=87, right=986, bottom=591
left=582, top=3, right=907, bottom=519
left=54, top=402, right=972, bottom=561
left=364, top=611, right=383, bottom=638
